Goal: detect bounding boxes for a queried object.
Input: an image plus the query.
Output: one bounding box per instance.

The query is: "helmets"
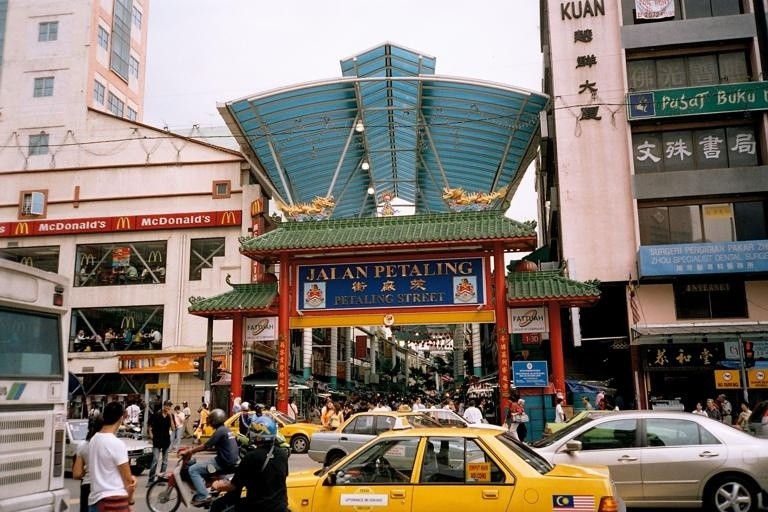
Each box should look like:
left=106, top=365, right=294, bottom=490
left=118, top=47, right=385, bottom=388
left=206, top=408, right=227, bottom=427
left=241, top=401, right=277, bottom=442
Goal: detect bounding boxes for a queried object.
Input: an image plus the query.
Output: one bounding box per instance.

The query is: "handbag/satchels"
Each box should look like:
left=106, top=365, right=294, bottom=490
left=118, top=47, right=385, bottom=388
left=512, top=414, right=528, bottom=423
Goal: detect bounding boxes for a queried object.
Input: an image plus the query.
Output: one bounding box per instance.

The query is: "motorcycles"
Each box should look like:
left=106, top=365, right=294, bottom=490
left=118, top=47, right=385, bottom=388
left=236, top=424, right=291, bottom=458
left=119, top=419, right=143, bottom=440
left=145, top=447, right=238, bottom=511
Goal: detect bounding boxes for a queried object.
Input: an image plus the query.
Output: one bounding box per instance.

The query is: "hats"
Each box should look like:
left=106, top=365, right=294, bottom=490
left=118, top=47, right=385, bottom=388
left=165, top=399, right=173, bottom=405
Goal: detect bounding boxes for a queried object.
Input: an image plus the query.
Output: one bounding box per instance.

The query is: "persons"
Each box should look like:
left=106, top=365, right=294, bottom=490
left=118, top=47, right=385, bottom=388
left=78, top=262, right=166, bottom=286
left=555, top=390, right=768, bottom=440
left=304, top=384, right=528, bottom=442
left=73, top=395, right=299, bottom=512
left=382, top=441, right=434, bottom=483
left=73, top=325, right=162, bottom=352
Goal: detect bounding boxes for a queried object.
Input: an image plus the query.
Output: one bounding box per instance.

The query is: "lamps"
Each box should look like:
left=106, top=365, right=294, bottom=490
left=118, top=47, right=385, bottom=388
left=356, top=120, right=374, bottom=195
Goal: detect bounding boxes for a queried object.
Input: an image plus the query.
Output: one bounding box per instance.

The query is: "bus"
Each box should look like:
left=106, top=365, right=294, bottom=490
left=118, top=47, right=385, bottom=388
left=0, top=258, right=73, bottom=511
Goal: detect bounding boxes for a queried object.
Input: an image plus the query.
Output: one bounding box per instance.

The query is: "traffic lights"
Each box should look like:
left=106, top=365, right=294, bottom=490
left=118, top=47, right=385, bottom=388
left=743, top=341, right=755, bottom=368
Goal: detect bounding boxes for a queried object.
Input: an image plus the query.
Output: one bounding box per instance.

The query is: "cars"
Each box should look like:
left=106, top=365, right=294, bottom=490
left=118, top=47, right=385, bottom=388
left=522, top=409, right=768, bottom=511
left=216, top=426, right=627, bottom=511
left=65, top=416, right=153, bottom=477
left=307, top=412, right=483, bottom=468
left=386, top=409, right=520, bottom=450
left=199, top=405, right=325, bottom=453
left=542, top=409, right=688, bottom=443
